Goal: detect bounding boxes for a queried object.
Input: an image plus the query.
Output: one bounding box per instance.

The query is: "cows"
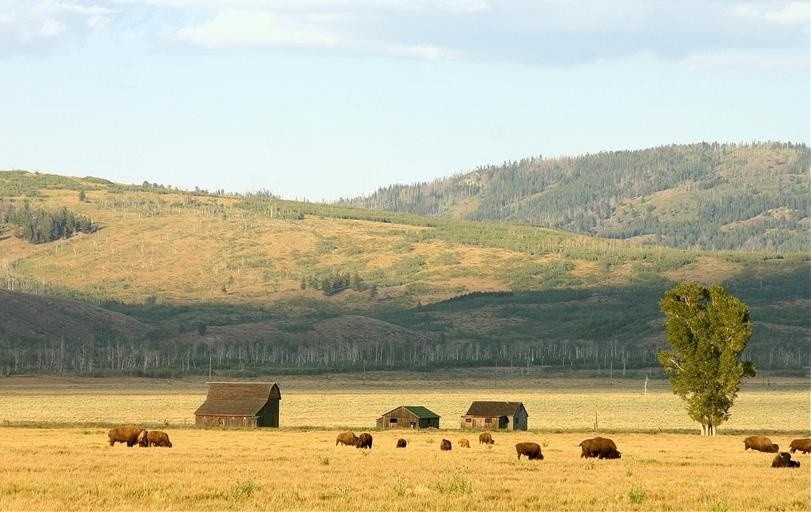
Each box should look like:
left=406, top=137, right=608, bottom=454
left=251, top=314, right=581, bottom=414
left=440, top=433, right=495, bottom=450
left=335, top=432, right=373, bottom=449
left=578, top=437, right=622, bottom=459
left=772, top=453, right=800, bottom=468
left=515, top=442, right=543, bottom=460
left=395, top=438, right=407, bottom=448
left=742, top=437, right=778, bottom=452
left=788, top=438, right=811, bottom=453
left=108, top=426, right=173, bottom=447
